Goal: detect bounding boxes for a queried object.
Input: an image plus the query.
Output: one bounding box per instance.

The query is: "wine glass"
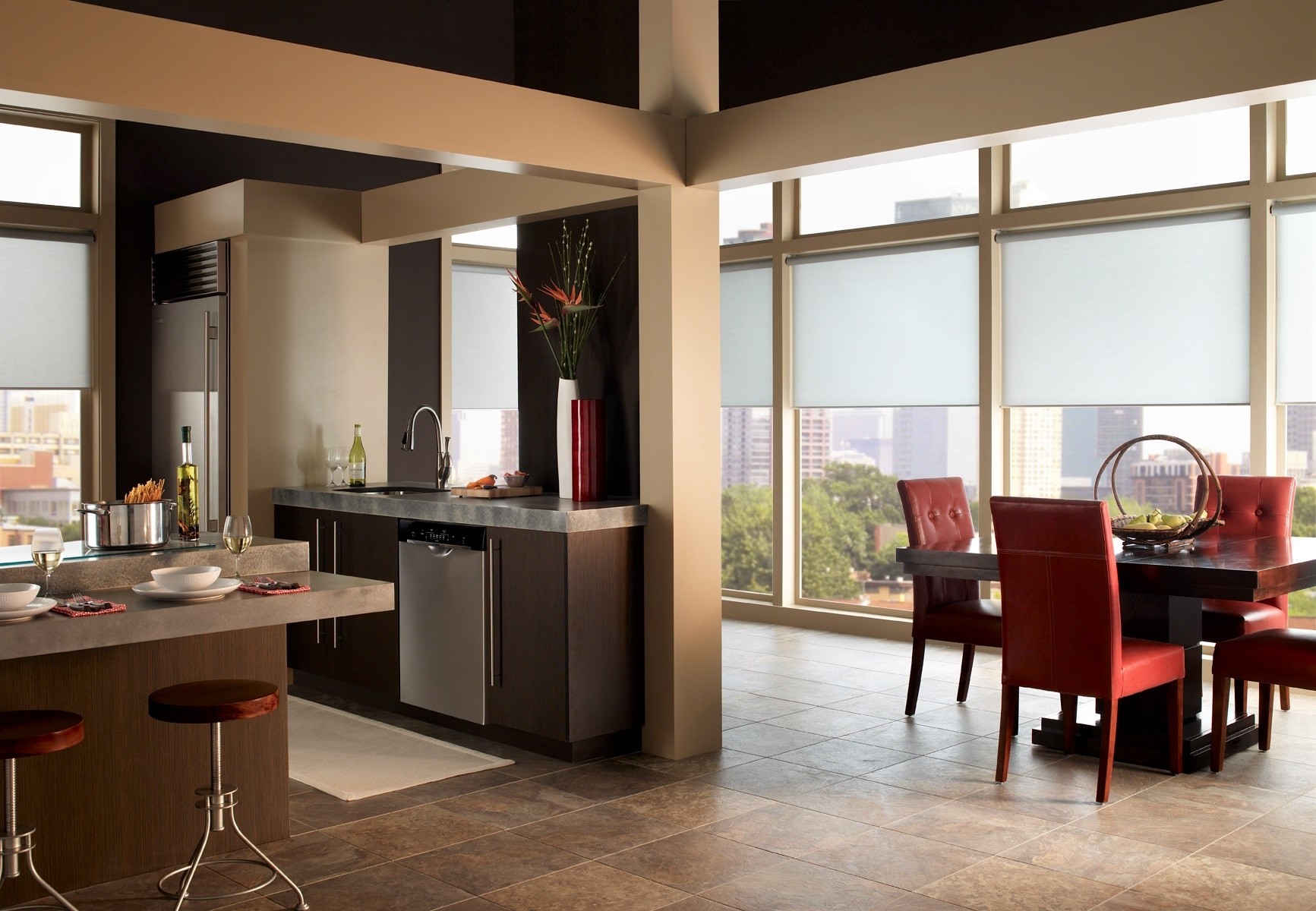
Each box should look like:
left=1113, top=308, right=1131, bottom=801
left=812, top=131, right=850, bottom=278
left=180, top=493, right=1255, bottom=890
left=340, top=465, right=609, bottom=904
left=223, top=515, right=253, bottom=585
left=324, top=447, right=338, bottom=487
left=31, top=528, right=67, bottom=604
left=335, top=447, right=350, bottom=486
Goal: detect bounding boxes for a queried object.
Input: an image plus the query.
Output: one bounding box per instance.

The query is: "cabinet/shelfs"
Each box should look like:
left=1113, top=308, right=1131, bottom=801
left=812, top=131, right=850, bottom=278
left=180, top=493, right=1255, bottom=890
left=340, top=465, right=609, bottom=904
left=484, top=527, right=642, bottom=764
left=270, top=505, right=398, bottom=713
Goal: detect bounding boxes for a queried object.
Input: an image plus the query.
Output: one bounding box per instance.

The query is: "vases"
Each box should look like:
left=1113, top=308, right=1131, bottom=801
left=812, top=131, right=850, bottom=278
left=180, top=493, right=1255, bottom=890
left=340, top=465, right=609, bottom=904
left=557, top=377, right=583, bottom=499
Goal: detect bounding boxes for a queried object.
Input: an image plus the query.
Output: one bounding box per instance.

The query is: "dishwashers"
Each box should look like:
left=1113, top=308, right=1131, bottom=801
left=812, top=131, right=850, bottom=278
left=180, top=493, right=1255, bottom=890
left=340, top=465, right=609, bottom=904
left=397, top=518, right=487, bottom=726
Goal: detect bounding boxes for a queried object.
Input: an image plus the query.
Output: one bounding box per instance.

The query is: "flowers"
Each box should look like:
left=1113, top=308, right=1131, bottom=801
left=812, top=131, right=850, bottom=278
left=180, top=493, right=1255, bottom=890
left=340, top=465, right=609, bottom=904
left=504, top=217, right=627, bottom=383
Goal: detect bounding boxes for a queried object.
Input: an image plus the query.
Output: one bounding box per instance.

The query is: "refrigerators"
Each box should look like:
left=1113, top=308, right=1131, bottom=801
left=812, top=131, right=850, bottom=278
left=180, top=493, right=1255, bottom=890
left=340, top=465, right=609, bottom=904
left=149, top=238, right=230, bottom=534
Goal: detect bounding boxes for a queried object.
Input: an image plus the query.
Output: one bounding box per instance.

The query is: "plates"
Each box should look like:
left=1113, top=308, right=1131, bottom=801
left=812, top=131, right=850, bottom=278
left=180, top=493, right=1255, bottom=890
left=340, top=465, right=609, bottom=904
left=131, top=578, right=242, bottom=601
left=0, top=597, right=58, bottom=624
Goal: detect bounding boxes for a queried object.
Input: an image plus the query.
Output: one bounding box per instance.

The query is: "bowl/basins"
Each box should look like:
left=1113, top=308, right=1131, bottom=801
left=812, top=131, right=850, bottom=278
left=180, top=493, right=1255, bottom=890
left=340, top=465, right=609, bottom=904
left=0, top=582, right=41, bottom=612
left=504, top=474, right=530, bottom=487
left=150, top=566, right=222, bottom=591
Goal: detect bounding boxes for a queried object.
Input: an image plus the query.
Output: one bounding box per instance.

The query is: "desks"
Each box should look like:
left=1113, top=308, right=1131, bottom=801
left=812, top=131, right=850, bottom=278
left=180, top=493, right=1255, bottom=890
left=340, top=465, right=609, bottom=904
left=896, top=536, right=1316, bottom=777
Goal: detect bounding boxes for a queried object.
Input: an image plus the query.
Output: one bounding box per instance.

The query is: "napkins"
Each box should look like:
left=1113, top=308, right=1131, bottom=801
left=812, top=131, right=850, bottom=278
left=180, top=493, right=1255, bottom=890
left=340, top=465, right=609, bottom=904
left=52, top=596, right=127, bottom=617
left=237, top=576, right=311, bottom=595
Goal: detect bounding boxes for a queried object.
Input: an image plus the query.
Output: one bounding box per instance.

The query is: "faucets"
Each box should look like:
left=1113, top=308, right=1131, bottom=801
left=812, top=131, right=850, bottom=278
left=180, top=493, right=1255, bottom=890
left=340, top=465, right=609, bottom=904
left=400, top=404, right=453, bottom=489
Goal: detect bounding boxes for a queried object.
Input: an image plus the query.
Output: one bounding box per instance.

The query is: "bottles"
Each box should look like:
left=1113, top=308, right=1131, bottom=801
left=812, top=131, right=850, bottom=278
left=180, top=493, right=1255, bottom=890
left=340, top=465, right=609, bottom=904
left=176, top=425, right=200, bottom=542
left=349, top=424, right=366, bottom=486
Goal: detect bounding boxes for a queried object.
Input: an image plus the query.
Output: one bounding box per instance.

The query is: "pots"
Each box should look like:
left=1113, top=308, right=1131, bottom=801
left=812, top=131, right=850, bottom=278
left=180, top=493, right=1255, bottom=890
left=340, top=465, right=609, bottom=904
left=73, top=498, right=178, bottom=552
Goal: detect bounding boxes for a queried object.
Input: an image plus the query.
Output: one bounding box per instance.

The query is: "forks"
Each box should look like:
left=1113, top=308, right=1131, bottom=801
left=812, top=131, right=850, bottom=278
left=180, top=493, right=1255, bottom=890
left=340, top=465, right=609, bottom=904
left=257, top=574, right=300, bottom=588
left=72, top=592, right=111, bottom=608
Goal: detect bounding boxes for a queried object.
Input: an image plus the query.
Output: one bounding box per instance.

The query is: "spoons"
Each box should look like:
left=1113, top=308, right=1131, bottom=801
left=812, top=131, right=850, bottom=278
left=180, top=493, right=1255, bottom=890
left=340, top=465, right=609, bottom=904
left=241, top=578, right=292, bottom=589
left=53, top=597, right=100, bottom=611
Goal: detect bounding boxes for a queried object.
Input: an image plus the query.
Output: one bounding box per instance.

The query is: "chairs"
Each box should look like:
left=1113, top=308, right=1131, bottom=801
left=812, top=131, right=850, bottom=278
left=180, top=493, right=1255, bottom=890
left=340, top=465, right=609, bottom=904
left=1193, top=474, right=1296, bottom=718
left=896, top=476, right=1002, bottom=717
left=1210, top=627, right=1316, bottom=773
left=989, top=496, right=1186, bottom=806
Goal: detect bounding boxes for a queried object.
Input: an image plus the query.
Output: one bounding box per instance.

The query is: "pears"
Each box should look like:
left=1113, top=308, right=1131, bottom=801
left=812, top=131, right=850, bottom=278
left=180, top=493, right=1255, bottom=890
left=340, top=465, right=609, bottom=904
left=1122, top=509, right=1208, bottom=529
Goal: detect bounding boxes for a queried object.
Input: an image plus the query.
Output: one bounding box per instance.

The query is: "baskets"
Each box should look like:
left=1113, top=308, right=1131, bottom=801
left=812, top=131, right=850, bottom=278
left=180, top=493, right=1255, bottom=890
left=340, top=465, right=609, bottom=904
left=1092, top=434, right=1221, bottom=547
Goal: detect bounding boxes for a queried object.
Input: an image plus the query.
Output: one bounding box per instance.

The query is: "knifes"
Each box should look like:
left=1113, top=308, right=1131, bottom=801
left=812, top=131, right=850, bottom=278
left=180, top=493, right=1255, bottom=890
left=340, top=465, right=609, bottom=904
left=483, top=485, right=507, bottom=490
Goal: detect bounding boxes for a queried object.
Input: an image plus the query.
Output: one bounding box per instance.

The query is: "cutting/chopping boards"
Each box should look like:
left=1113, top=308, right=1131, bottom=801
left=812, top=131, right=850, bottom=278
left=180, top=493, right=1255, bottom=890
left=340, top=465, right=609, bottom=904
left=451, top=487, right=543, bottom=499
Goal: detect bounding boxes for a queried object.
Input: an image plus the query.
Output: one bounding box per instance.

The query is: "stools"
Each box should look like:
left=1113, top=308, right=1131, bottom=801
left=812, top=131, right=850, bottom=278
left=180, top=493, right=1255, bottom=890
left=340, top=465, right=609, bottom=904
left=0, top=710, right=85, bottom=911
left=148, top=679, right=311, bottom=911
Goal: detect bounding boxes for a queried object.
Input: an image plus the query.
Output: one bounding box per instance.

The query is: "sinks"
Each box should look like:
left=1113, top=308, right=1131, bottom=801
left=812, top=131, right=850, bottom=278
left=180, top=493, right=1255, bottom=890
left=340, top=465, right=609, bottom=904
left=360, top=491, right=427, bottom=495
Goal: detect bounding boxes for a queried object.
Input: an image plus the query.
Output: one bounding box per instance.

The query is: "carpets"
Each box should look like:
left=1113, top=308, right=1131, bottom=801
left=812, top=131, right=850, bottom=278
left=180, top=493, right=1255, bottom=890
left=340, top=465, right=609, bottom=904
left=287, top=694, right=516, bottom=802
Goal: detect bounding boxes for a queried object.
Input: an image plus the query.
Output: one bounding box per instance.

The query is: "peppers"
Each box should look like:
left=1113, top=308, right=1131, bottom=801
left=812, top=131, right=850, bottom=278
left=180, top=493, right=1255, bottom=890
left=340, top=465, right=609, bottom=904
left=467, top=470, right=527, bottom=488
